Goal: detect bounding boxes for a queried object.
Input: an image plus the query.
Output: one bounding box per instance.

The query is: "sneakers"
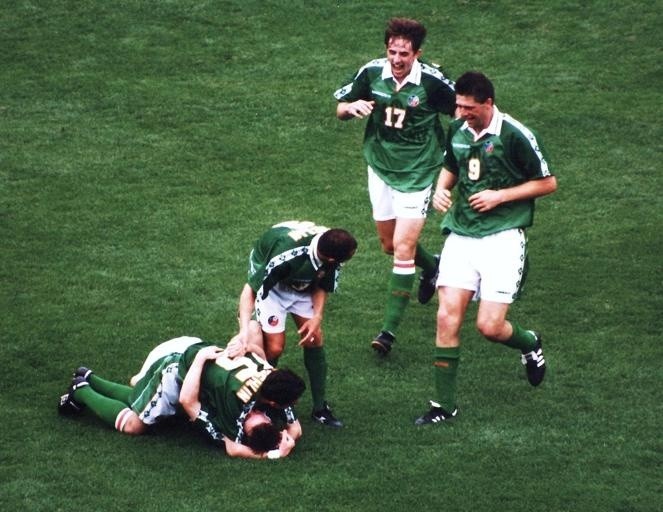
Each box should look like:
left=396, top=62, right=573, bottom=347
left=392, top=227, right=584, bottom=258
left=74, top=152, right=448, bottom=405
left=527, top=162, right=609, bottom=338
left=311, top=400, right=344, bottom=427
left=418, top=254, right=441, bottom=304
left=370, top=329, right=396, bottom=354
left=520, top=330, right=546, bottom=387
left=414, top=399, right=458, bottom=425
left=58, top=366, right=94, bottom=413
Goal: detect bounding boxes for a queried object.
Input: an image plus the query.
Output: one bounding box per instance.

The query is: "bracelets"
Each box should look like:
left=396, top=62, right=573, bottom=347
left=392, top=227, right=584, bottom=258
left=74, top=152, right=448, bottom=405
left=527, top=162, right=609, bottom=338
left=268, top=449, right=280, bottom=460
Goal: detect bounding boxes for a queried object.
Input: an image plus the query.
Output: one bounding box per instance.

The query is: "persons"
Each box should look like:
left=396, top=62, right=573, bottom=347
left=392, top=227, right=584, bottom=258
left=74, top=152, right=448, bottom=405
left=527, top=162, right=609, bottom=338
left=412, top=71, right=557, bottom=425
left=226, top=220, right=357, bottom=427
left=131, top=337, right=281, bottom=453
left=331, top=20, right=459, bottom=353
left=61, top=366, right=302, bottom=446
